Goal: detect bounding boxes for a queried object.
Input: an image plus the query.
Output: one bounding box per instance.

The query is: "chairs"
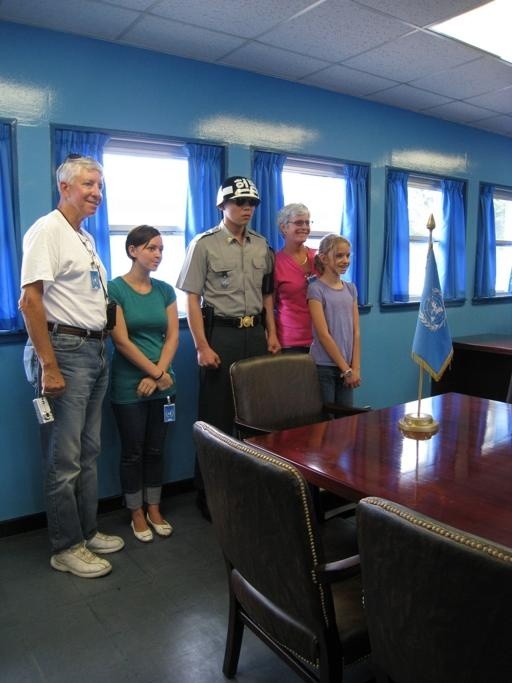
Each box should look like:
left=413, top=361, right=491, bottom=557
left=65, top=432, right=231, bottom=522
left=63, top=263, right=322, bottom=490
left=356, top=496, right=512, bottom=682
left=229, top=352, right=373, bottom=440
left=192, top=419, right=373, bottom=682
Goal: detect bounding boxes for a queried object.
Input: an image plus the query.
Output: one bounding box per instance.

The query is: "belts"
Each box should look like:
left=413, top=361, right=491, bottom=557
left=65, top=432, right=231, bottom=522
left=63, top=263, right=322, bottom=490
left=44, top=321, right=108, bottom=342
left=209, top=314, right=264, bottom=328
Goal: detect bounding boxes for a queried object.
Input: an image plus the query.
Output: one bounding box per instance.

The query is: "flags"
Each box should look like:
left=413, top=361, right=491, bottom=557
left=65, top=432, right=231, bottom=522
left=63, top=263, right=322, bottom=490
left=18, top=155, right=124, bottom=573
left=414, top=245, right=455, bottom=381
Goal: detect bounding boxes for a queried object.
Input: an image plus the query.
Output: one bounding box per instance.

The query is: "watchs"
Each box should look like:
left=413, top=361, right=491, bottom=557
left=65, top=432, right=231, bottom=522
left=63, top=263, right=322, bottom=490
left=340, top=368, right=352, bottom=378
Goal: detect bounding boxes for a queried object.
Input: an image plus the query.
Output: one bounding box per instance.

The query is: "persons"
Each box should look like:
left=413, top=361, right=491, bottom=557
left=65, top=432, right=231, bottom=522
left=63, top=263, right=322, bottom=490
left=306, top=234, right=360, bottom=420
left=106, top=225, right=179, bottom=540
left=175, top=176, right=281, bottom=523
left=270, top=204, right=323, bottom=353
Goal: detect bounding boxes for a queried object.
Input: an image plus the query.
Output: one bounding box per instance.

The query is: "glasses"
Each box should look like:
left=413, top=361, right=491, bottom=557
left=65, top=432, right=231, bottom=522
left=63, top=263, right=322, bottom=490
left=285, top=220, right=313, bottom=227
left=231, top=198, right=259, bottom=207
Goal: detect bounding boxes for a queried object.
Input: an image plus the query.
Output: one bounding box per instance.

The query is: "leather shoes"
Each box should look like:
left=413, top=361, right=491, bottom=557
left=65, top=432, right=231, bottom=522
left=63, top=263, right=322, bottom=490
left=131, top=519, right=153, bottom=543
left=147, top=514, right=173, bottom=536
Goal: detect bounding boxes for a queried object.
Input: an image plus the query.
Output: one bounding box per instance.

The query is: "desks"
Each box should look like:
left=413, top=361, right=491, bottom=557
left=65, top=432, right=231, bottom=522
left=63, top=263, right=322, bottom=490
left=241, top=392, right=512, bottom=570
left=431, top=332, right=512, bottom=404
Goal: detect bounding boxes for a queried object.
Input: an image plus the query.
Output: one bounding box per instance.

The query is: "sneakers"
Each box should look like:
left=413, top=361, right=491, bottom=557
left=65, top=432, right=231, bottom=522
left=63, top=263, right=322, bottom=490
left=50, top=541, right=112, bottom=578
left=88, top=532, right=124, bottom=554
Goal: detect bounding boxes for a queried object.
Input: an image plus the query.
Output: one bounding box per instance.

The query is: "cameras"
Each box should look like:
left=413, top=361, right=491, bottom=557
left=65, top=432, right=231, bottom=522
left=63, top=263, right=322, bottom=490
left=33, top=397, right=55, bottom=425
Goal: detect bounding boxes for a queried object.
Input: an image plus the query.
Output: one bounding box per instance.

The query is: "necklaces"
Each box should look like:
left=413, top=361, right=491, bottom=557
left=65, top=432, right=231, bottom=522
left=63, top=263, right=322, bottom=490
left=297, top=257, right=308, bottom=267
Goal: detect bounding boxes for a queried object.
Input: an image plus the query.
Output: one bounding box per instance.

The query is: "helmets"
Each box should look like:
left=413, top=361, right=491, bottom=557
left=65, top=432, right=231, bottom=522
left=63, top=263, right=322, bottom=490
left=216, top=176, right=260, bottom=206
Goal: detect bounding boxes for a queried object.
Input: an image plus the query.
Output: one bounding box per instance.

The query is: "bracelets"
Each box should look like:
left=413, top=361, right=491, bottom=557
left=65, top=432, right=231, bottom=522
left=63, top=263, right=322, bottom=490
left=155, top=370, right=164, bottom=381
